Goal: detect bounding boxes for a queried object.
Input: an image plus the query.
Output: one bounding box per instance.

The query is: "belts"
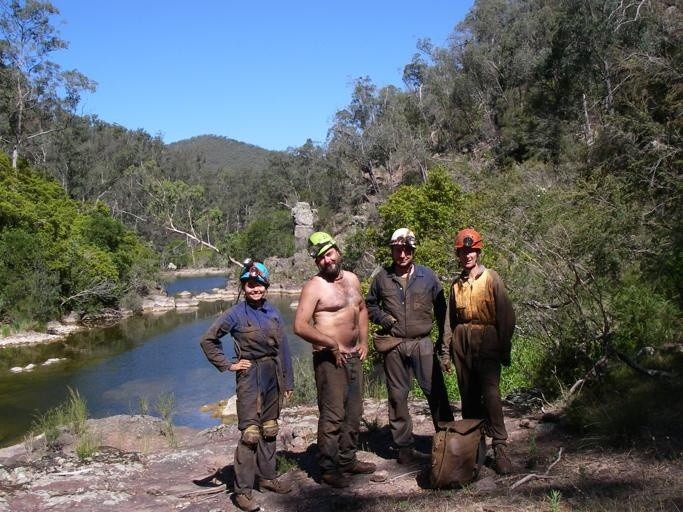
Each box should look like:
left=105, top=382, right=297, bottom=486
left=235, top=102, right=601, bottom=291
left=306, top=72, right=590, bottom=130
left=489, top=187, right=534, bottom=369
left=343, top=353, right=359, bottom=359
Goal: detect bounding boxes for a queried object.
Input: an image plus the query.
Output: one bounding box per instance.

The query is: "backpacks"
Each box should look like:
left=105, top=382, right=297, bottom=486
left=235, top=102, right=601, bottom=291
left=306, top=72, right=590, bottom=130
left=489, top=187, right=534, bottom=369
left=430, top=419, right=485, bottom=489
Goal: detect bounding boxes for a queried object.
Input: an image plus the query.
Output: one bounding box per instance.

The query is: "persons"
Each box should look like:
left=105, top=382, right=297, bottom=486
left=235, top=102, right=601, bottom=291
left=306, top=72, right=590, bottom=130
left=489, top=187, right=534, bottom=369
left=199, top=261, right=296, bottom=511
left=292, top=231, right=376, bottom=488
left=442, top=227, right=516, bottom=477
left=364, top=227, right=456, bottom=465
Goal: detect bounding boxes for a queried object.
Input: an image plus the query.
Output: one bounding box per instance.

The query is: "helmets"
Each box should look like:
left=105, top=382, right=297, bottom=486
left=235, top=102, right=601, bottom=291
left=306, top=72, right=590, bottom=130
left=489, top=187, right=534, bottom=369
left=240, top=262, right=270, bottom=287
left=308, top=232, right=336, bottom=257
left=456, top=229, right=484, bottom=250
left=389, top=228, right=416, bottom=249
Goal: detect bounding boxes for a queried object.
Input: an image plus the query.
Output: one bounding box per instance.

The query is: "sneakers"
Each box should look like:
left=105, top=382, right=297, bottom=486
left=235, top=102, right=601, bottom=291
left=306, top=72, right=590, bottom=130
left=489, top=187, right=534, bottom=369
left=399, top=447, right=431, bottom=464
left=337, top=460, right=376, bottom=474
left=259, top=479, right=289, bottom=493
left=235, top=493, right=260, bottom=512
left=321, top=473, right=351, bottom=487
left=493, top=444, right=512, bottom=475
